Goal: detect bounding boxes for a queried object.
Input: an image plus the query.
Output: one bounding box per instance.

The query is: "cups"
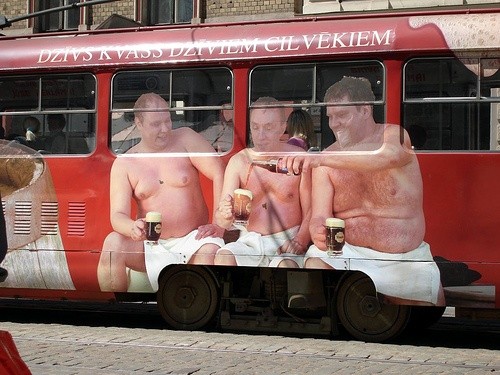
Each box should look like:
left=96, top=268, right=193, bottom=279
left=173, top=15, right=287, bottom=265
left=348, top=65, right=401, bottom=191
left=140, top=213, right=163, bottom=242
left=231, top=189, right=253, bottom=225
left=321, top=220, right=345, bottom=256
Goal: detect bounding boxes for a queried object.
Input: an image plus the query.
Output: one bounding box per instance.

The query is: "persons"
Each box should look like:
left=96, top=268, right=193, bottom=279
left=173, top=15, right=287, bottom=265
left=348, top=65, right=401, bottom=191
left=95, top=93, right=226, bottom=294
left=20, top=115, right=43, bottom=141
left=219, top=97, right=314, bottom=270
left=284, top=110, right=315, bottom=150
left=47, top=114, right=66, bottom=137
left=281, top=79, right=447, bottom=306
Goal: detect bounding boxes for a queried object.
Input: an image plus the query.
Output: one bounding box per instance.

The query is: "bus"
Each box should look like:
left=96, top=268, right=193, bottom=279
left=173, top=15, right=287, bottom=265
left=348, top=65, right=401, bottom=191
left=0, top=3, right=499, bottom=344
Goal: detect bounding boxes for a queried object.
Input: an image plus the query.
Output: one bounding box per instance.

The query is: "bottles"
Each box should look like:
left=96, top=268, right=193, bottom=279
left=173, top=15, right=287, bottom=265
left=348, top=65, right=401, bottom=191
left=251, top=159, right=303, bottom=176
